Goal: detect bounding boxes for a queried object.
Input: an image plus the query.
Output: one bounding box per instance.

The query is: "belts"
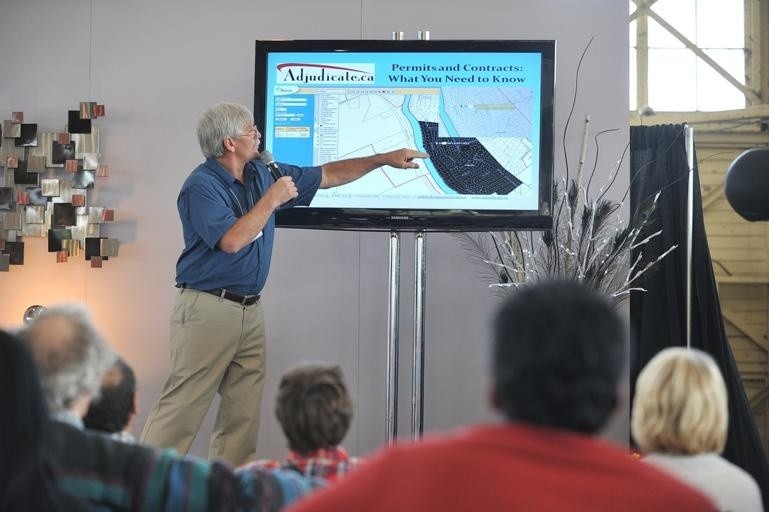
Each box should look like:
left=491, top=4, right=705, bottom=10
left=176, top=282, right=261, bottom=306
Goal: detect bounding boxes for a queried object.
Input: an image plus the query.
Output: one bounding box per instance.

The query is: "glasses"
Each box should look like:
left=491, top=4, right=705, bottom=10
left=241, top=125, right=258, bottom=138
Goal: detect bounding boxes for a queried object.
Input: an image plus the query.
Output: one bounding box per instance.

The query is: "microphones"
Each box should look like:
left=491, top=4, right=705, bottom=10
left=258, top=151, right=281, bottom=183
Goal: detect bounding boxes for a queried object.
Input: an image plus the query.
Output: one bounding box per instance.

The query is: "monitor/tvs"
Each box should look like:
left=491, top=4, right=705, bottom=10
left=248, top=40, right=557, bottom=233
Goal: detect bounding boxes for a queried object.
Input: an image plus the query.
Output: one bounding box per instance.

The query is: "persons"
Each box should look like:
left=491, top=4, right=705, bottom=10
left=139, top=102, right=429, bottom=472
left=288, top=281, right=715, bottom=510
left=235, top=364, right=364, bottom=486
left=630, top=346, right=762, bottom=511
left=2, top=307, right=283, bottom=512
